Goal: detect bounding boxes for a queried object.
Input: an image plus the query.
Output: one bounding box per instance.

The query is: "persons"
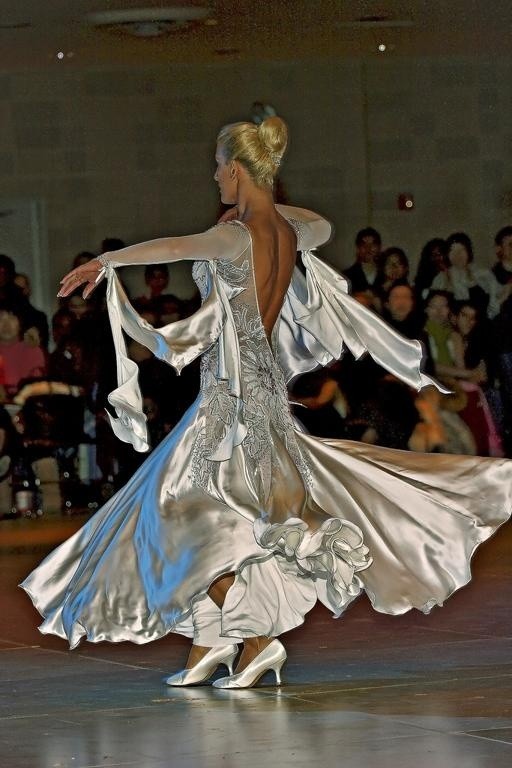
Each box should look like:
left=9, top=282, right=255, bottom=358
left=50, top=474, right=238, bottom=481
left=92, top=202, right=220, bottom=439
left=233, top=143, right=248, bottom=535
left=1, top=237, right=202, bottom=518
left=288, top=222, right=511, bottom=462
left=55, top=115, right=299, bottom=690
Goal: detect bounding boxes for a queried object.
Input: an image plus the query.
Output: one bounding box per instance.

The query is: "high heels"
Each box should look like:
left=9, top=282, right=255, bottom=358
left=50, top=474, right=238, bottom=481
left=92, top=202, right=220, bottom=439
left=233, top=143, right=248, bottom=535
left=211, top=639, right=288, bottom=690
left=165, top=639, right=239, bottom=687
